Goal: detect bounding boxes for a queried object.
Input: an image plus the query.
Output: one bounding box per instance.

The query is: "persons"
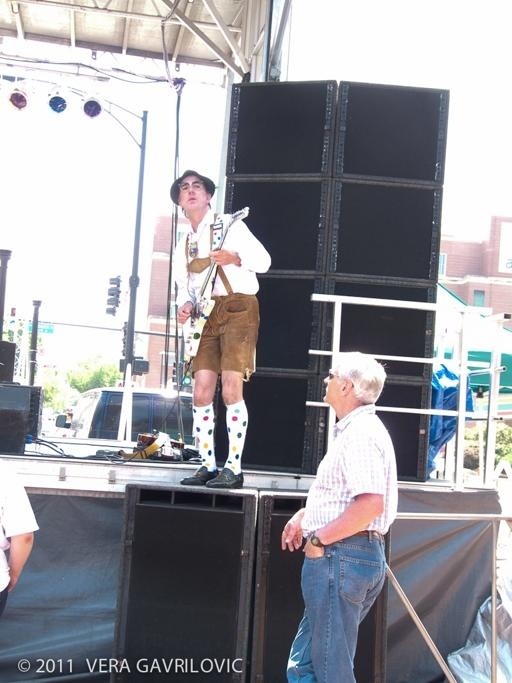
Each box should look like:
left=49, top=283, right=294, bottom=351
left=280, top=352, right=399, bottom=683
left=170, top=169, right=271, bottom=488
left=0, top=458, right=40, bottom=614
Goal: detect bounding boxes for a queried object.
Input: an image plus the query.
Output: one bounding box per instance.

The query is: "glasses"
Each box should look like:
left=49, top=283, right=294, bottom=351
left=178, top=182, right=202, bottom=191
left=329, top=373, right=335, bottom=380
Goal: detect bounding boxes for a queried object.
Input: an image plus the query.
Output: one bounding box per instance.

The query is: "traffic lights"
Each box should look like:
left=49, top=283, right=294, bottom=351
left=182, top=362, right=192, bottom=386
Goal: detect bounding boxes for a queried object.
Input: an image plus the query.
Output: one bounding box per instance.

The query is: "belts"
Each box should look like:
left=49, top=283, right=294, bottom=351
left=357, top=529, right=386, bottom=541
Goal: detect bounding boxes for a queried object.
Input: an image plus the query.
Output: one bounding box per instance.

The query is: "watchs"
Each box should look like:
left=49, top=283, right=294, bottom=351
left=309, top=531, right=328, bottom=550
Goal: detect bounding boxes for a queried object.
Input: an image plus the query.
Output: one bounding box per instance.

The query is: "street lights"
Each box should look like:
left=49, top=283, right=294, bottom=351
left=1, top=73, right=149, bottom=439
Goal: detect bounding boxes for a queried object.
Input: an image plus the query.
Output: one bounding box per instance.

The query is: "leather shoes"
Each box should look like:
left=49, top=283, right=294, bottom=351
left=179, top=466, right=243, bottom=488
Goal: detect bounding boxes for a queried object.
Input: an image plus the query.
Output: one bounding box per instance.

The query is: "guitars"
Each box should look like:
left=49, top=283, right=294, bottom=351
left=187, top=207, right=250, bottom=357
left=137, top=431, right=184, bottom=460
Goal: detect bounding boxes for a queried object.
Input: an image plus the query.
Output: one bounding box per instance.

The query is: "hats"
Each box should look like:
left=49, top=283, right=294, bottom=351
left=170, top=169, right=216, bottom=205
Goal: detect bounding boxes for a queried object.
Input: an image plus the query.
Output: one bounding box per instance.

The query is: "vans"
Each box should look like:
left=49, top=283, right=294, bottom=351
left=56, top=387, right=193, bottom=442
